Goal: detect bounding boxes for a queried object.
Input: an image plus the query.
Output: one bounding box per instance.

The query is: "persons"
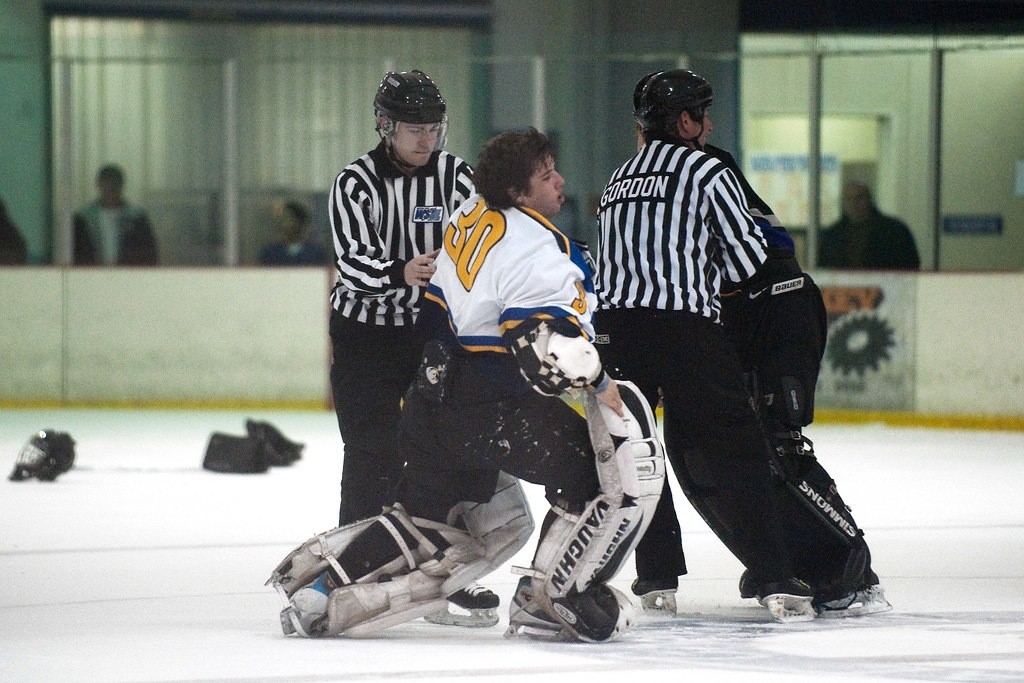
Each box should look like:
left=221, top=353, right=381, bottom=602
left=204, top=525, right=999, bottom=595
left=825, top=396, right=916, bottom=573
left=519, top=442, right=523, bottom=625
left=262, top=133, right=665, bottom=643
left=818, top=176, right=919, bottom=271
left=636, top=123, right=893, bottom=618
left=591, top=69, right=822, bottom=623
left=70, top=166, right=157, bottom=267
left=328, top=68, right=500, bottom=628
left=259, top=201, right=328, bottom=266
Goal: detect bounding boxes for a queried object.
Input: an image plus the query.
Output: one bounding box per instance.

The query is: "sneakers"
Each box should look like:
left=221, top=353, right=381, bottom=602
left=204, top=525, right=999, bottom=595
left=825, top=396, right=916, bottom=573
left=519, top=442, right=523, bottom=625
left=813, top=560, right=893, bottom=617
left=424, top=578, right=499, bottom=627
left=631, top=572, right=679, bottom=615
left=280, top=570, right=341, bottom=639
left=753, top=573, right=817, bottom=622
left=501, top=576, right=577, bottom=641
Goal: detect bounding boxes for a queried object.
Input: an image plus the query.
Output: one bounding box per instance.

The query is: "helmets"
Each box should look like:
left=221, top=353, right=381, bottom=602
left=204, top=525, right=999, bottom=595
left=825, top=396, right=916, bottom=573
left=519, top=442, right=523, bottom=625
left=630, top=72, right=664, bottom=117
left=638, top=69, right=715, bottom=119
left=372, top=69, right=445, bottom=125
left=7, top=429, right=75, bottom=483
